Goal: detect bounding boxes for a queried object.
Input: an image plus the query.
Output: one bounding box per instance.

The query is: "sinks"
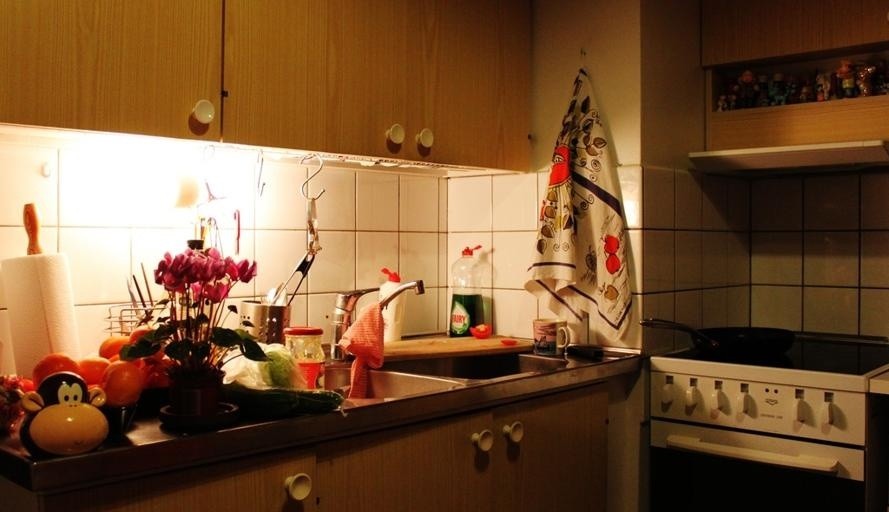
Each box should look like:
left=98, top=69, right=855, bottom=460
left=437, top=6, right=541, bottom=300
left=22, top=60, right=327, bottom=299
left=371, top=348, right=571, bottom=381
left=295, top=361, right=469, bottom=402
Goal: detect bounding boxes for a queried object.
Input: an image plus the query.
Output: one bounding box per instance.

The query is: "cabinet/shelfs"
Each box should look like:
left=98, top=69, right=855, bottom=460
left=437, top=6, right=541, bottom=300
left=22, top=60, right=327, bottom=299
left=221, top=1, right=537, bottom=180
left=314, top=379, right=610, bottom=512
left=0, top=444, right=314, bottom=512
left=0, top=1, right=221, bottom=145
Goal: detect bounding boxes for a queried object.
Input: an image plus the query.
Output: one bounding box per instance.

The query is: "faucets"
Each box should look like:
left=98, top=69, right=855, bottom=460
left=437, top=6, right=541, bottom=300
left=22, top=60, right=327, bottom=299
left=329, top=278, right=426, bottom=363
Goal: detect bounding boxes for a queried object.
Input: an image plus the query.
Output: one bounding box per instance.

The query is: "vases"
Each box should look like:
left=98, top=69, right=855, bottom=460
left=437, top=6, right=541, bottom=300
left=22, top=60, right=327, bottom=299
left=169, top=369, right=225, bottom=414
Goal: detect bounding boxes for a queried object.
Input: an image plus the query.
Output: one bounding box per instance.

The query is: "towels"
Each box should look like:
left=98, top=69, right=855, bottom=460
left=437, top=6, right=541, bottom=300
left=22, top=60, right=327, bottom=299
left=523, top=65, right=634, bottom=343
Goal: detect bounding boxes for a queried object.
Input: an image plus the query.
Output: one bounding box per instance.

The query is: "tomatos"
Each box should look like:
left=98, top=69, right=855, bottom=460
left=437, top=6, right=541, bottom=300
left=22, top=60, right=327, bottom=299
left=470, top=323, right=492, bottom=338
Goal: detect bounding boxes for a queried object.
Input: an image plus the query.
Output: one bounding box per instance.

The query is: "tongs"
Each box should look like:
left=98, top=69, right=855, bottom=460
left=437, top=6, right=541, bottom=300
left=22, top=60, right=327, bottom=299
left=270, top=249, right=315, bottom=307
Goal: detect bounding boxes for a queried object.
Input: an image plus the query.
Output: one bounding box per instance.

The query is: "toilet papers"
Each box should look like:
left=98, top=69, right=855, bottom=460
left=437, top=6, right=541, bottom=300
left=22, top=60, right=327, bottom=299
left=1, top=252, right=83, bottom=381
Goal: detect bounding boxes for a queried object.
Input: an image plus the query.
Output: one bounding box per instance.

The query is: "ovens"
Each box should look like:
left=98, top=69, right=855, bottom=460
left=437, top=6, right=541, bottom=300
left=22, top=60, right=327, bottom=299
left=646, top=421, right=889, bottom=512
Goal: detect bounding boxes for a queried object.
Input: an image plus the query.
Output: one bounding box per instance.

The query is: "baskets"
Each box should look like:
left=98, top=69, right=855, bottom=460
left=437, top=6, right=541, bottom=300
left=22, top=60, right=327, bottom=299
left=107, top=304, right=166, bottom=337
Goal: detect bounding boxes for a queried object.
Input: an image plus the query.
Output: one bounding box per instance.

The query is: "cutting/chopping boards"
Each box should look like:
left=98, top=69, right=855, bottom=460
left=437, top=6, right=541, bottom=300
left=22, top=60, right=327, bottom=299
left=383, top=335, right=534, bottom=364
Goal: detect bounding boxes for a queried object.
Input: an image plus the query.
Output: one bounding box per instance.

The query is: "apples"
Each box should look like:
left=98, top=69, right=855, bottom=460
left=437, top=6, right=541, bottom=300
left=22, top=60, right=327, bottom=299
left=600, top=235, right=619, bottom=254
left=606, top=254, right=621, bottom=275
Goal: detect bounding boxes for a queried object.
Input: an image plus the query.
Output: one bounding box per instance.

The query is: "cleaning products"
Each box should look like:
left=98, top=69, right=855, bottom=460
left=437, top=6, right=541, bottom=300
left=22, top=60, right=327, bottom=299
left=375, top=264, right=407, bottom=341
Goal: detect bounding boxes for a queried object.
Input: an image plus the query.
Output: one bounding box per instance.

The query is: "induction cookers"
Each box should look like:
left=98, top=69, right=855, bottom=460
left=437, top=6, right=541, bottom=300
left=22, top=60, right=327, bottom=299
left=650, top=333, right=889, bottom=437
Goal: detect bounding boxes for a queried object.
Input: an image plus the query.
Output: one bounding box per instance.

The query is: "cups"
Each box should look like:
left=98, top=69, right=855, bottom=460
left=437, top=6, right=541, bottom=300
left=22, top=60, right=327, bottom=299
left=533, top=318, right=570, bottom=357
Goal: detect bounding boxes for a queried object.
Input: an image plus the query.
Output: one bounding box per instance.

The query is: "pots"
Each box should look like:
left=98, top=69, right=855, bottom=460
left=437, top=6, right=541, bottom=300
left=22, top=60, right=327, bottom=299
left=639, top=317, right=799, bottom=356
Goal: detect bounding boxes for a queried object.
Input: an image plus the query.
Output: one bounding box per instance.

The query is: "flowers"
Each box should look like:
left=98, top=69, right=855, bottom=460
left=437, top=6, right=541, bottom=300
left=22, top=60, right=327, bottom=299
left=118, top=247, right=274, bottom=371
